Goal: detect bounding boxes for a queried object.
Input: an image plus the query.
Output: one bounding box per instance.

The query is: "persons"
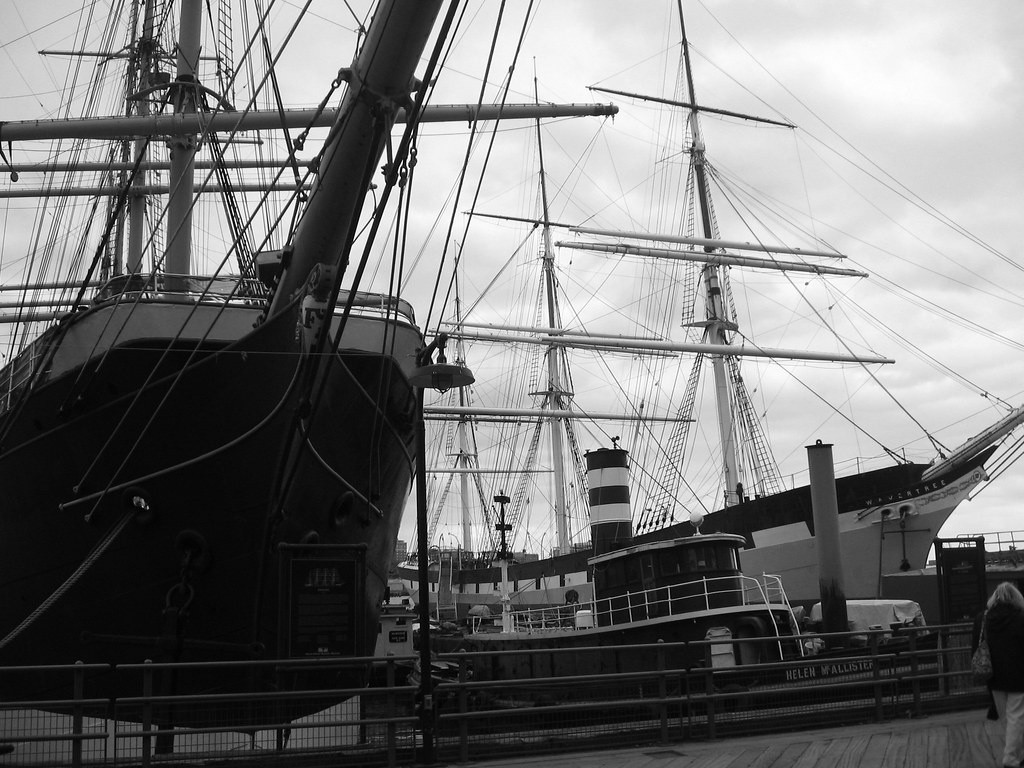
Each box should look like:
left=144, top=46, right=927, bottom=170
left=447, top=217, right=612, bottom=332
left=971, top=582, right=1024, bottom=768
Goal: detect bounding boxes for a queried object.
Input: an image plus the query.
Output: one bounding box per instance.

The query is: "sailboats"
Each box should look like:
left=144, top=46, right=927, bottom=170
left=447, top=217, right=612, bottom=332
left=0, top=0, right=620, bottom=728
left=396, top=0, right=1024, bottom=609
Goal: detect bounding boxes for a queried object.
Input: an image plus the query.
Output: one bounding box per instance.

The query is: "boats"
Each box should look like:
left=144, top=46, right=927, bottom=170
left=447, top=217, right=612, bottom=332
left=424, top=508, right=812, bottom=705
left=368, top=587, right=417, bottom=681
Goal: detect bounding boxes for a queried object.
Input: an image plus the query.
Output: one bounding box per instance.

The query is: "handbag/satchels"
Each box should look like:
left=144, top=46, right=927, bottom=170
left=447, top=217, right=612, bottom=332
left=972, top=641, right=992, bottom=678
